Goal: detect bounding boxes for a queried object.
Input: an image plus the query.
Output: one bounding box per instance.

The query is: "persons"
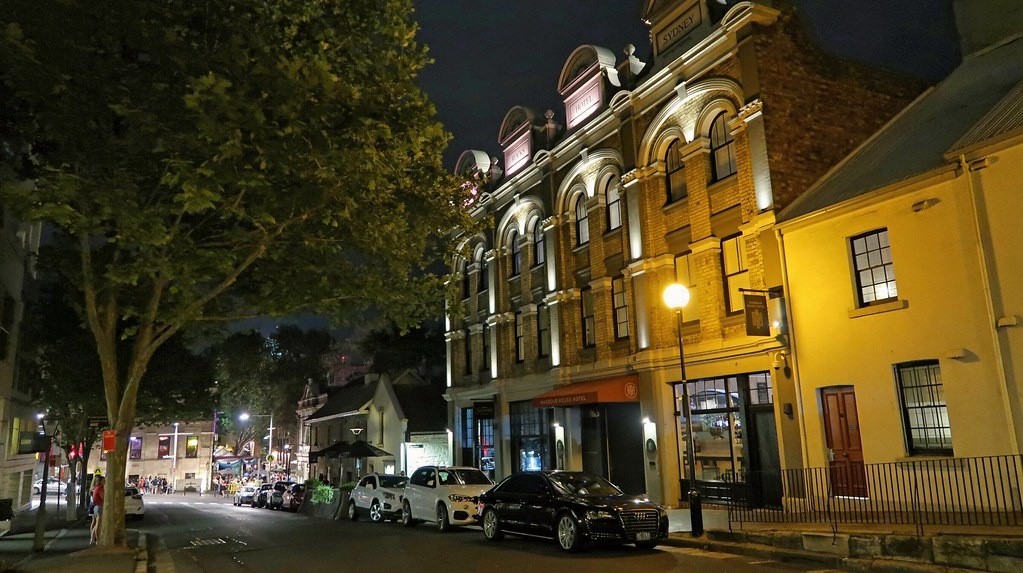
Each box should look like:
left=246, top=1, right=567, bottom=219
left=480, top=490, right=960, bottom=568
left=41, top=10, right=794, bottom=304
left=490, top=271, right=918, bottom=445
left=525, top=446, right=540, bottom=468
left=214, top=461, right=286, bottom=499
left=400, top=470, right=405, bottom=477
left=88, top=475, right=106, bottom=545
left=140, top=475, right=168, bottom=494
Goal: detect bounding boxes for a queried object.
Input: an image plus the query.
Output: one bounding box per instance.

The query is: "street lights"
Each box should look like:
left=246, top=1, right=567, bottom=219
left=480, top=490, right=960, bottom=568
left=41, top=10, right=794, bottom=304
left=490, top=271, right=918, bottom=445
left=663, top=281, right=707, bottom=538
left=208, top=410, right=226, bottom=490
left=172, top=423, right=179, bottom=494
left=239, top=412, right=273, bottom=453
left=31, top=418, right=61, bottom=551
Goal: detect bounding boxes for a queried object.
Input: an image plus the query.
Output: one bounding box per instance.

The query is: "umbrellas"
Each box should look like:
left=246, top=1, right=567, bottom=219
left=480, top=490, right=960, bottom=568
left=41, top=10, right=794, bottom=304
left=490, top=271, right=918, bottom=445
left=309, top=441, right=394, bottom=485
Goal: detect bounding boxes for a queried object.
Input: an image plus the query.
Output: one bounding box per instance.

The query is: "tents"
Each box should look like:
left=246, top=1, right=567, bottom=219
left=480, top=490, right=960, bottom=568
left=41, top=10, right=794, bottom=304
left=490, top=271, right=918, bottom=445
left=217, top=458, right=256, bottom=479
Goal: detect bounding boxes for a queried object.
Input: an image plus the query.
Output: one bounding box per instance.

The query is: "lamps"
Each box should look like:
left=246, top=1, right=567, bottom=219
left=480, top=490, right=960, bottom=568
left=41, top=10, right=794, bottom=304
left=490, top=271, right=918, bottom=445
left=946, top=347, right=965, bottom=360
left=911, top=201, right=929, bottom=212
left=350, top=428, right=364, bottom=439
left=971, top=157, right=989, bottom=171
left=997, top=315, right=1017, bottom=328
left=784, top=402, right=793, bottom=415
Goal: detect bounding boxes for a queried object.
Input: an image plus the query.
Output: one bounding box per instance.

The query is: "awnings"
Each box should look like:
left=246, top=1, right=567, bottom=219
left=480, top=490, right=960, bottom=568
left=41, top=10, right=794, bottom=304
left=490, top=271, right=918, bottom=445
left=529, top=374, right=640, bottom=407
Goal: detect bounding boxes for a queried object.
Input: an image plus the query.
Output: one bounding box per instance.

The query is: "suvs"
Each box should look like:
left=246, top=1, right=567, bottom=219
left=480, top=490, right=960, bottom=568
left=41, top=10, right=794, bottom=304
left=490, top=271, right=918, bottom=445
left=347, top=472, right=428, bottom=524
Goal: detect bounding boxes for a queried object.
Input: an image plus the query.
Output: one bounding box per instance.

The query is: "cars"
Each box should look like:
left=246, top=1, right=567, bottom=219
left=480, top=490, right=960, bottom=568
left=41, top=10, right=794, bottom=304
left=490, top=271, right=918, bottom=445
left=68, top=473, right=104, bottom=494
left=476, top=471, right=670, bottom=552
left=33, top=478, right=67, bottom=494
left=125, top=486, right=146, bottom=520
left=280, top=483, right=305, bottom=511
left=233, top=485, right=256, bottom=506
left=267, top=481, right=298, bottom=509
left=676, top=388, right=740, bottom=412
left=251, top=483, right=273, bottom=508
left=401, top=466, right=498, bottom=534
